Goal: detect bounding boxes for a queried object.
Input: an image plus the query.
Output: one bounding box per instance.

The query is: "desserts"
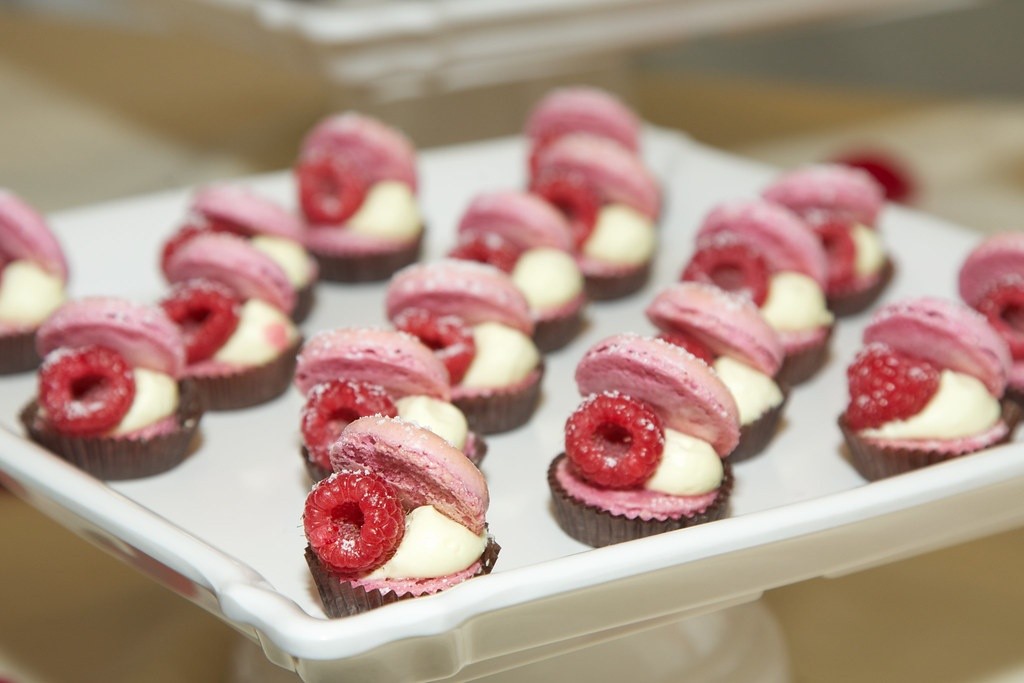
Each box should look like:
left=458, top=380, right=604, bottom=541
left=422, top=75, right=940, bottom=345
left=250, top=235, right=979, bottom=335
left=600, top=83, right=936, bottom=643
left=0, top=80, right=1024, bottom=627
